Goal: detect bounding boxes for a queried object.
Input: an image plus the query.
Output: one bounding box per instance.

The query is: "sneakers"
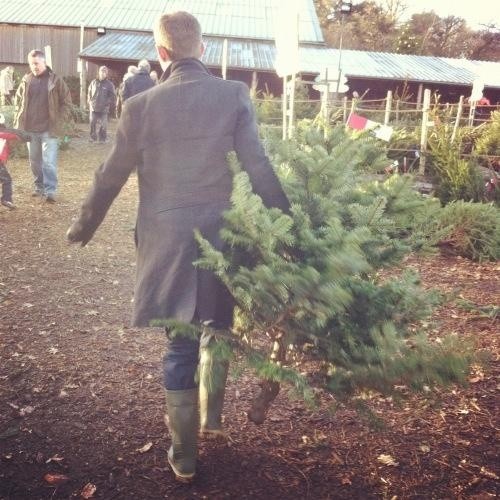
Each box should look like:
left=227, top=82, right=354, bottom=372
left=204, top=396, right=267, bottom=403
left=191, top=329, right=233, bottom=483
left=1, top=200, right=16, bottom=210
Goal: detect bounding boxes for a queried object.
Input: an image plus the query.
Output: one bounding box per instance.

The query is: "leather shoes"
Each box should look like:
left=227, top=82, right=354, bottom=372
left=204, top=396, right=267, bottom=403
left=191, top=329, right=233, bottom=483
left=46, top=192, right=56, bottom=204
left=31, top=189, right=40, bottom=197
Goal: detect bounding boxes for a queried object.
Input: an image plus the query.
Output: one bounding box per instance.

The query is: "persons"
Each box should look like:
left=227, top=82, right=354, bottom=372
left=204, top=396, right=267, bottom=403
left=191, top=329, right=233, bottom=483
left=66, top=11, right=294, bottom=485
left=88, top=60, right=158, bottom=143
left=0, top=115, right=18, bottom=211
left=13, top=51, right=74, bottom=204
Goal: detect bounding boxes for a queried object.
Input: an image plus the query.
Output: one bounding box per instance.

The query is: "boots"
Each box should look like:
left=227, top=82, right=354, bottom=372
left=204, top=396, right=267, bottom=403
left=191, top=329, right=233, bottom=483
left=166, top=386, right=201, bottom=484
left=199, top=343, right=230, bottom=435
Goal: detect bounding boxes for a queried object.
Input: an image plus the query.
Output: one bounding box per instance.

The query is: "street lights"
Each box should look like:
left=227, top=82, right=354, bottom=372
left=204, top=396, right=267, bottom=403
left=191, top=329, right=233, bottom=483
left=97, top=26, right=107, bottom=79
left=334, top=3, right=352, bottom=99
